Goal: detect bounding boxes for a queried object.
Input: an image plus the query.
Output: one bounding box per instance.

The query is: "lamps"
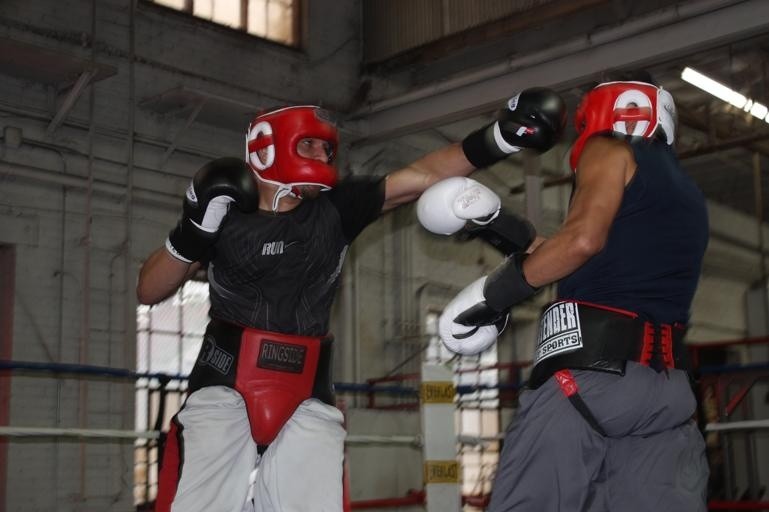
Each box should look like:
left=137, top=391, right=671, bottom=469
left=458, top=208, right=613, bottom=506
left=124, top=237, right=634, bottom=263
left=682, top=65, right=769, bottom=123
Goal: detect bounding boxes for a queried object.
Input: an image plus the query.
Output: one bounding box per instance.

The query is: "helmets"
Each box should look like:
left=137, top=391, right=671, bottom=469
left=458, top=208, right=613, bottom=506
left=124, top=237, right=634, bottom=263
left=245, top=106, right=337, bottom=197
left=569, top=80, right=678, bottom=174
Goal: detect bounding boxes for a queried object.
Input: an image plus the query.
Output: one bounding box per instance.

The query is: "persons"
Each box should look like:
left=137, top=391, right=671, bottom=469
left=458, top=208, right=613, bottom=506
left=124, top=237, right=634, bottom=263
left=138, top=85, right=568, bottom=512
left=417, top=68, right=709, bottom=512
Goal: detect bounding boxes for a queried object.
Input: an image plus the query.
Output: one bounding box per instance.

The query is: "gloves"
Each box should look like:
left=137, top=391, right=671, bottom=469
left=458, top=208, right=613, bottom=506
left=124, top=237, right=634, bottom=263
left=438, top=252, right=541, bottom=356
left=463, top=86, right=565, bottom=169
left=166, top=157, right=258, bottom=264
left=416, top=177, right=536, bottom=258
